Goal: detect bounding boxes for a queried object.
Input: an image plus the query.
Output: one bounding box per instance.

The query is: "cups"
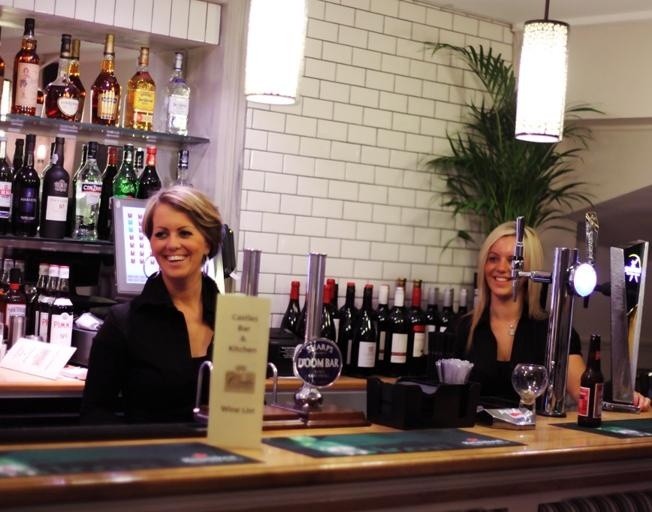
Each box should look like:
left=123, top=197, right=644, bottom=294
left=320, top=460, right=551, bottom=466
left=511, top=363, right=549, bottom=416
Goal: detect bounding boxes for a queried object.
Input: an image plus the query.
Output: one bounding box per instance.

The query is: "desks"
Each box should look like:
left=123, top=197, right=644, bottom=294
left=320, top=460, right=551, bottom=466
left=0, top=402, right=652, bottom=512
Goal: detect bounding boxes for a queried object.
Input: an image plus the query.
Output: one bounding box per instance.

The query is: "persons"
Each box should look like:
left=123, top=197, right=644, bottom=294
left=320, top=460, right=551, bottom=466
left=441, top=220, right=651, bottom=413
left=80, top=184, right=225, bottom=421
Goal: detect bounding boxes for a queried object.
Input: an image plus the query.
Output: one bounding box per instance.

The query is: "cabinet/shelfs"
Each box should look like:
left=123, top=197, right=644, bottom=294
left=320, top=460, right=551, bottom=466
left=263, top=378, right=368, bottom=417
left=0, top=0, right=246, bottom=445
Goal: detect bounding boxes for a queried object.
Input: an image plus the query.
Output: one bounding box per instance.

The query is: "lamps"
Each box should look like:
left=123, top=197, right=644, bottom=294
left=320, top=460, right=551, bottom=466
left=243, top=0, right=309, bottom=105
left=514, top=1, right=572, bottom=145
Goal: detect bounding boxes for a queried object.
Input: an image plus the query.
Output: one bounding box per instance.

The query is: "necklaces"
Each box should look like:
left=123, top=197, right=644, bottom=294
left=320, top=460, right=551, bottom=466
left=485, top=306, right=523, bottom=336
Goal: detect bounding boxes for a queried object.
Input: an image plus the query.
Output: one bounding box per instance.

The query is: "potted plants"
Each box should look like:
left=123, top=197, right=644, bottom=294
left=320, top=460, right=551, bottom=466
left=416, top=36, right=606, bottom=287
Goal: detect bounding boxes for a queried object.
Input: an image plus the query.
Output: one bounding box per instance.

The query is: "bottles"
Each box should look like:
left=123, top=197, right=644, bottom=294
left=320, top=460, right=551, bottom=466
left=30, top=264, right=48, bottom=344
left=99, top=147, right=117, bottom=240
left=113, top=144, right=139, bottom=198
left=14, top=262, right=25, bottom=275
left=12, top=18, right=40, bottom=116
left=40, top=142, right=56, bottom=205
left=376, top=285, right=390, bottom=369
left=125, top=46, right=156, bottom=131
left=68, top=39, right=86, bottom=121
left=352, top=284, right=376, bottom=378
left=322, top=285, right=336, bottom=341
left=440, top=288, right=455, bottom=332
left=89, top=34, right=120, bottom=126
left=12, top=133, right=38, bottom=238
left=6, top=268, right=26, bottom=337
left=407, top=279, right=426, bottom=376
left=39, top=263, right=59, bottom=345
left=281, top=281, right=300, bottom=331
left=0, top=27, right=5, bottom=101
left=134, top=150, right=145, bottom=180
left=0, top=260, right=14, bottom=329
left=173, top=151, right=193, bottom=187
left=162, top=52, right=189, bottom=135
left=385, top=286, right=410, bottom=374
left=426, top=286, right=438, bottom=350
left=73, top=145, right=87, bottom=203
left=139, top=148, right=162, bottom=199
left=327, top=279, right=340, bottom=343
left=579, top=334, right=604, bottom=428
left=0, top=136, right=11, bottom=236
left=341, top=284, right=358, bottom=375
left=46, top=34, right=79, bottom=121
left=456, top=290, right=468, bottom=319
left=75, top=142, right=103, bottom=240
left=48, top=266, right=74, bottom=347
left=42, top=137, right=68, bottom=238
left=13, top=138, right=24, bottom=172
